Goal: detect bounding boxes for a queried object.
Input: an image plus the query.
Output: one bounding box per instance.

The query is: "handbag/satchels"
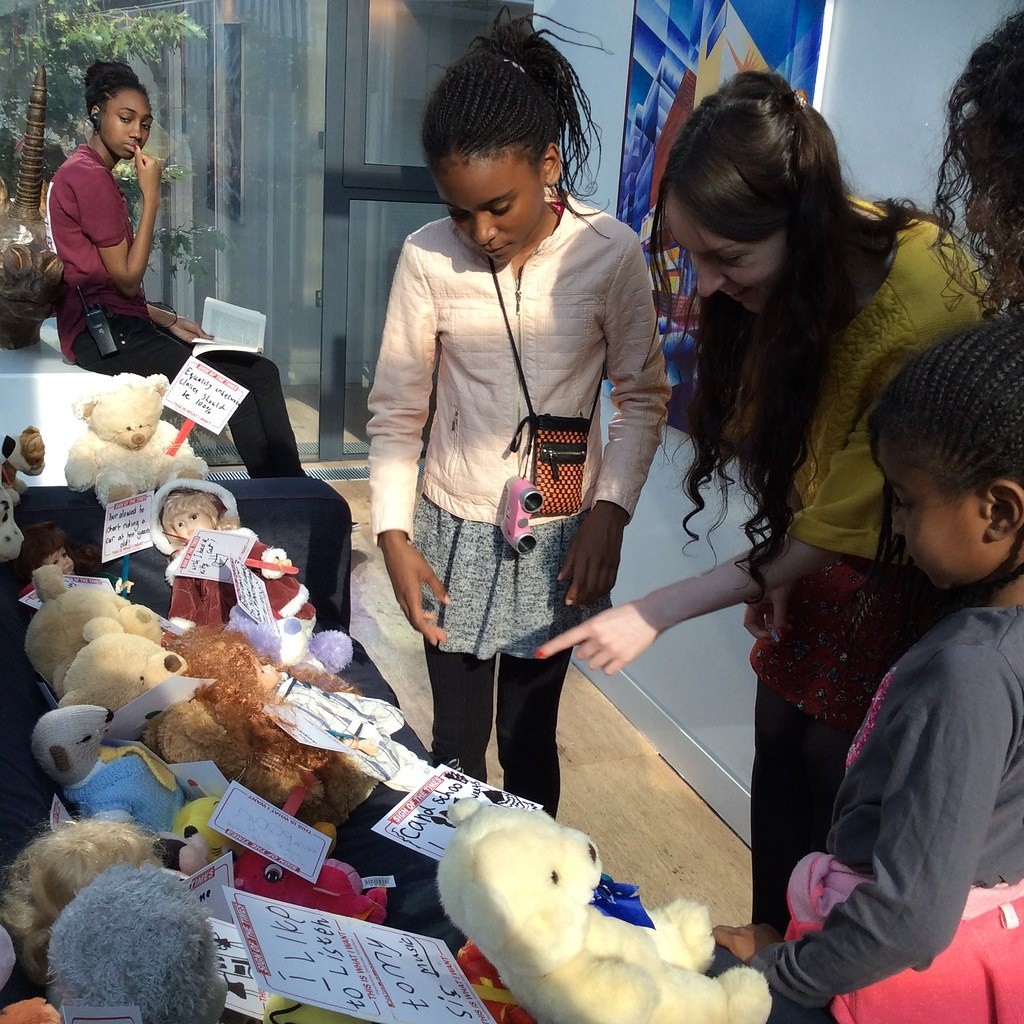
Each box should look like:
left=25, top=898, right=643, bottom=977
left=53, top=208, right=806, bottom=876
left=511, top=414, right=591, bottom=519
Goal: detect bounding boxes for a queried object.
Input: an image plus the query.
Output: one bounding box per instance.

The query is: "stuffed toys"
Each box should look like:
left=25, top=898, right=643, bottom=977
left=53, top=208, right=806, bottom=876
left=0, top=370, right=773, bottom=1024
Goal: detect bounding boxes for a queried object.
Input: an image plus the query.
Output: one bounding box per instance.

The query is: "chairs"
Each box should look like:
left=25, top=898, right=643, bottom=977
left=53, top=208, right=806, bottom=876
left=0, top=478, right=476, bottom=1024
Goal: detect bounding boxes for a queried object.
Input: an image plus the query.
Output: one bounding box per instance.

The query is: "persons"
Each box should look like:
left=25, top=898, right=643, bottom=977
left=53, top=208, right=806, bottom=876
left=366, top=18, right=670, bottom=816
left=46, top=59, right=305, bottom=478
left=537, top=18, right=1024, bottom=1024
left=29, top=530, right=132, bottom=602
left=160, top=488, right=315, bottom=634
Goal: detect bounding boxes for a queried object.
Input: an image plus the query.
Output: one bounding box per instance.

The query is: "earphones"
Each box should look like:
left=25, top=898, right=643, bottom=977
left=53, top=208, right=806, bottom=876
left=91, top=112, right=101, bottom=130
